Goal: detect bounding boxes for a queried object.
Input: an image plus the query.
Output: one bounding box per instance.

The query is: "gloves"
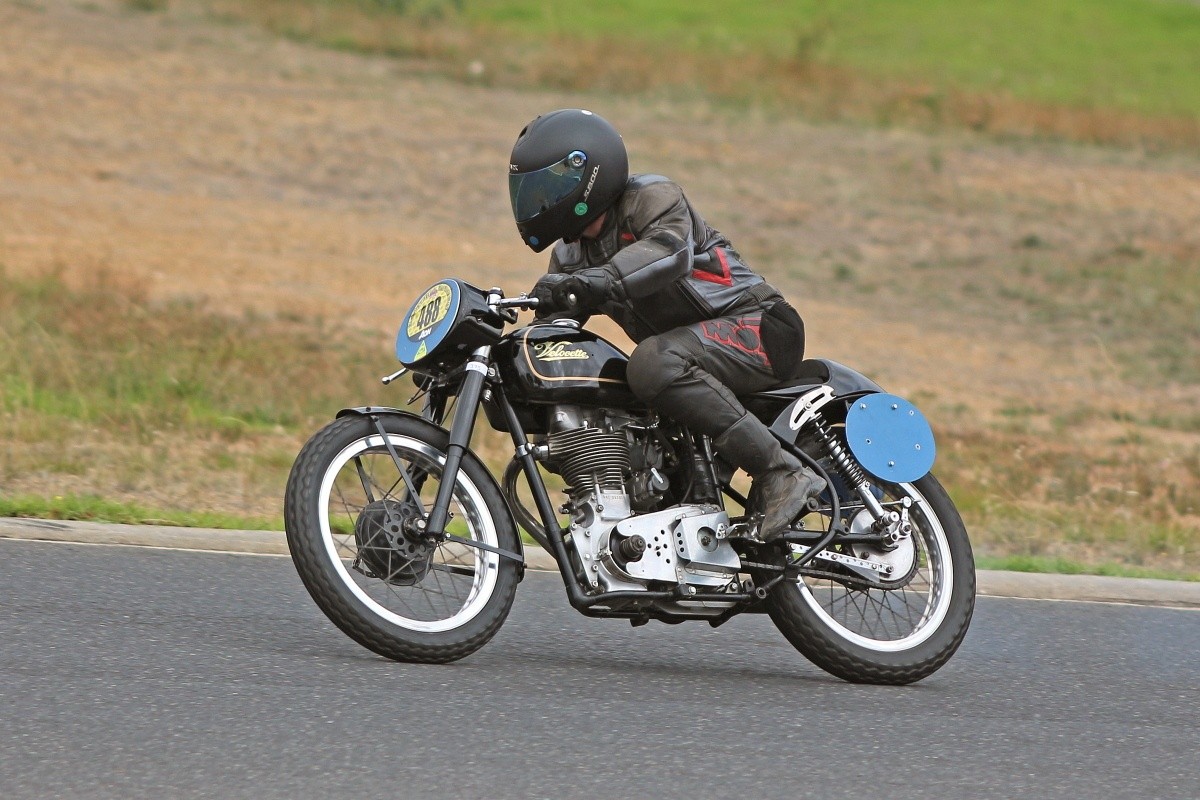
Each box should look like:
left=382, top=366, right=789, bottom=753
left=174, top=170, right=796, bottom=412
left=520, top=274, right=590, bottom=320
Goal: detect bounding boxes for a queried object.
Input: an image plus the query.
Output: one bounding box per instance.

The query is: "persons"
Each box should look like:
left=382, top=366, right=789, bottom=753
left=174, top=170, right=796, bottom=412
left=507, top=107, right=829, bottom=542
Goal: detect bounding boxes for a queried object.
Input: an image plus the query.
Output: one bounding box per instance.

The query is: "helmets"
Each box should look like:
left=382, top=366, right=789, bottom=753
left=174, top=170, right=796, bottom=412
left=507, top=108, right=632, bottom=254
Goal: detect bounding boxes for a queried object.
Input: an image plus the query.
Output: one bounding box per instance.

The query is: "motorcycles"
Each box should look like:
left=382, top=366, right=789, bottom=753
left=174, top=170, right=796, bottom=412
left=280, top=277, right=978, bottom=687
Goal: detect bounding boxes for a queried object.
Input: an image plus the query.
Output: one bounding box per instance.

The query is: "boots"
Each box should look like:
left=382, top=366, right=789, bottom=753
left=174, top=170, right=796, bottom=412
left=712, top=410, right=826, bottom=540
